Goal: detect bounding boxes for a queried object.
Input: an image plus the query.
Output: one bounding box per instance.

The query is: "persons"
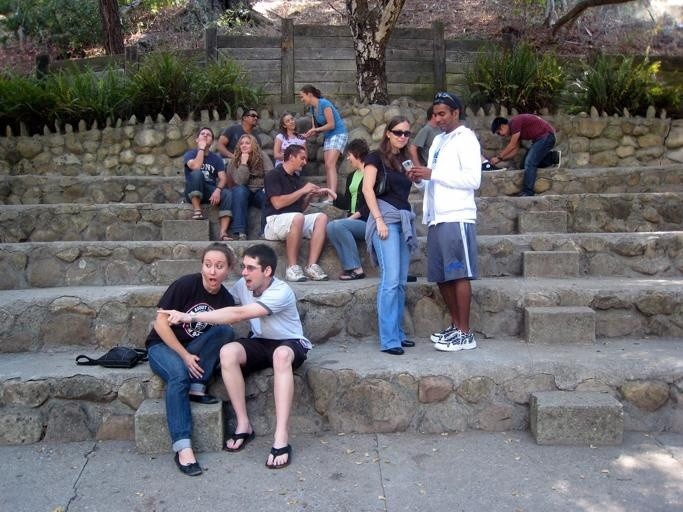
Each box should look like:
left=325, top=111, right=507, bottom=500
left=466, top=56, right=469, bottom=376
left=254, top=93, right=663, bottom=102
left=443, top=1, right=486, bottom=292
left=183, top=127, right=233, bottom=240
left=226, top=134, right=274, bottom=241
left=143, top=242, right=236, bottom=472
left=410, top=103, right=509, bottom=173
left=406, top=91, right=482, bottom=351
left=260, top=144, right=328, bottom=282
left=490, top=114, right=561, bottom=197
left=300, top=84, right=348, bottom=203
left=272, top=112, right=308, bottom=177
left=214, top=109, right=273, bottom=172
left=360, top=115, right=415, bottom=355
left=311, top=139, right=370, bottom=280
left=158, top=243, right=313, bottom=469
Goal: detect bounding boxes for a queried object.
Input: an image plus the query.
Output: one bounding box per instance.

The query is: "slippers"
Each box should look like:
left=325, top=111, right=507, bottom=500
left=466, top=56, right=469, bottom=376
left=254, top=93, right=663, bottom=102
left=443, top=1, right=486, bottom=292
left=267, top=444, right=291, bottom=469
left=223, top=430, right=255, bottom=452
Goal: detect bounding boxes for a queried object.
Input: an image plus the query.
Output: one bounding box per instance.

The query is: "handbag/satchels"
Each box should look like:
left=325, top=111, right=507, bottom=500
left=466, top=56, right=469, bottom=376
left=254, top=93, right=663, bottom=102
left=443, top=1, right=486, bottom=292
left=374, top=169, right=391, bottom=195
left=101, top=345, right=139, bottom=368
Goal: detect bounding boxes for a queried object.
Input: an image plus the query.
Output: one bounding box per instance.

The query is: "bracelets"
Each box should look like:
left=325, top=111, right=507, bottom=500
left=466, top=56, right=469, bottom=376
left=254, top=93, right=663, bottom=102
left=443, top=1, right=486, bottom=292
left=215, top=186, right=223, bottom=190
left=190, top=313, right=196, bottom=323
left=199, top=148, right=205, bottom=152
left=497, top=153, right=503, bottom=161
left=374, top=215, right=382, bottom=219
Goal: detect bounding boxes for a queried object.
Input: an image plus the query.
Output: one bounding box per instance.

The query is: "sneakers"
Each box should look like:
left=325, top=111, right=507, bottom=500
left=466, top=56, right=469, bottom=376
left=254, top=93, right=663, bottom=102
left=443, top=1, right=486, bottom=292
left=554, top=151, right=562, bottom=168
left=430, top=324, right=459, bottom=342
left=188, top=393, right=218, bottom=404
left=285, top=264, right=306, bottom=281
left=517, top=190, right=532, bottom=196
left=304, top=264, right=329, bottom=280
left=174, top=452, right=201, bottom=476
left=482, top=161, right=507, bottom=172
left=435, top=331, right=478, bottom=352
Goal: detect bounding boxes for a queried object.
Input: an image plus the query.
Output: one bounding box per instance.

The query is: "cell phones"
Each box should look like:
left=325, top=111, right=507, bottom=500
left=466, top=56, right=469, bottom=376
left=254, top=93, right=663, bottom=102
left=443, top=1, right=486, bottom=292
left=402, top=160, right=414, bottom=172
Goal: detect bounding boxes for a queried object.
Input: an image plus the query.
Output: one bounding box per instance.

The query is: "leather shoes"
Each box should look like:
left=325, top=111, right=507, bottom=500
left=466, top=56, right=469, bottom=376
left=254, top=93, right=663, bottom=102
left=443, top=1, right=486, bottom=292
left=386, top=339, right=414, bottom=355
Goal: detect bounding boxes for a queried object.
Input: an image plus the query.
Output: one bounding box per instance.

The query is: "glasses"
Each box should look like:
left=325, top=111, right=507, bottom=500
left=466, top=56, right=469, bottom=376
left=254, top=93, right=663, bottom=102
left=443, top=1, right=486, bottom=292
left=239, top=263, right=262, bottom=272
left=388, top=128, right=411, bottom=137
left=435, top=91, right=456, bottom=104
left=247, top=113, right=260, bottom=119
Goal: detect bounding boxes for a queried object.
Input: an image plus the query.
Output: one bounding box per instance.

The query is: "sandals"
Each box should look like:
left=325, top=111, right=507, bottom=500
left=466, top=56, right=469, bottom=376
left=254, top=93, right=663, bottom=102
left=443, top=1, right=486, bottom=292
left=192, top=209, right=204, bottom=220
left=340, top=267, right=365, bottom=280
left=219, top=235, right=247, bottom=240
